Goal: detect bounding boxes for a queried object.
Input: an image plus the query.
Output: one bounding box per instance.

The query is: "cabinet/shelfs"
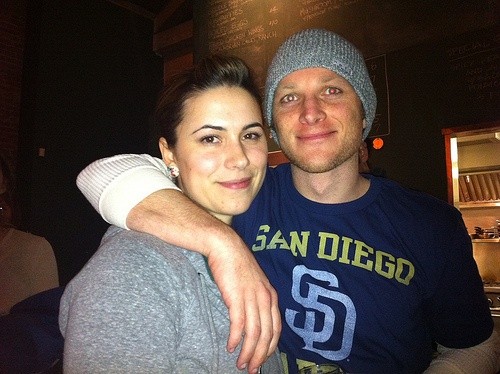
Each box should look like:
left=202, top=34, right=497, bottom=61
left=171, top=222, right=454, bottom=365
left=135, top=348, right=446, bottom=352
left=441, top=119, right=500, bottom=314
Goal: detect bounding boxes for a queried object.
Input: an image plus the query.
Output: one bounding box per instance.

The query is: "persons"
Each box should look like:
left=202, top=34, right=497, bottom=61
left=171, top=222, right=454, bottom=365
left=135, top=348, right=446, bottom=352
left=58, top=55, right=286, bottom=374
left=0, top=159, right=65, bottom=374
left=76, top=28, right=500, bottom=374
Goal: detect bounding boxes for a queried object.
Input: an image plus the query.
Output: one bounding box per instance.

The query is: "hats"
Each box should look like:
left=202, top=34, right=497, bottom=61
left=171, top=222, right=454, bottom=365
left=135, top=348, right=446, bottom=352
left=263, top=28, right=378, bottom=149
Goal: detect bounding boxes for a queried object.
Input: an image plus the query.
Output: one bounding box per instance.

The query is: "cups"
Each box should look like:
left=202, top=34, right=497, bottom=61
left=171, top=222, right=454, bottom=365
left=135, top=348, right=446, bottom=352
left=296, top=364, right=346, bottom=374
